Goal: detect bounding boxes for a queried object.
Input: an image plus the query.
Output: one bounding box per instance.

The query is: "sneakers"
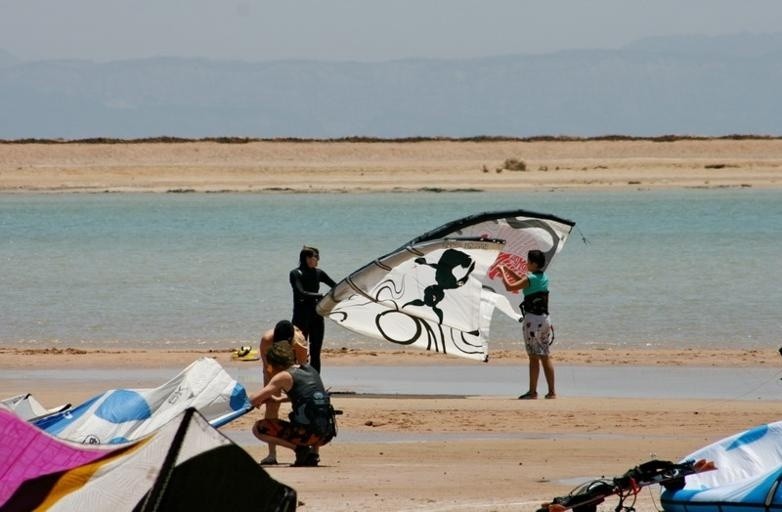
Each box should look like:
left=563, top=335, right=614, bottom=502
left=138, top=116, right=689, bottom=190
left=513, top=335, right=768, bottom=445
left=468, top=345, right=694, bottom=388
left=288, top=444, right=320, bottom=467
left=260, top=455, right=278, bottom=465
left=519, top=391, right=559, bottom=399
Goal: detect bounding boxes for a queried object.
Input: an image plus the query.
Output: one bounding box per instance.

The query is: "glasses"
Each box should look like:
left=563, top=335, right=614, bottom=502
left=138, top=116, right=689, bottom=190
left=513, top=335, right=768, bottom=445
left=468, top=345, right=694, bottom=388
left=307, top=253, right=319, bottom=258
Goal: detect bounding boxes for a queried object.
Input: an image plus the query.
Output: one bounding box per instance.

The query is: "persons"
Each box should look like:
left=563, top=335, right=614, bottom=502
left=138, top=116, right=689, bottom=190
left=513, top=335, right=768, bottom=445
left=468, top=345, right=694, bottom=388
left=496, top=248, right=557, bottom=400
left=260, top=320, right=310, bottom=466
left=243, top=339, right=338, bottom=468
left=289, top=245, right=339, bottom=377
